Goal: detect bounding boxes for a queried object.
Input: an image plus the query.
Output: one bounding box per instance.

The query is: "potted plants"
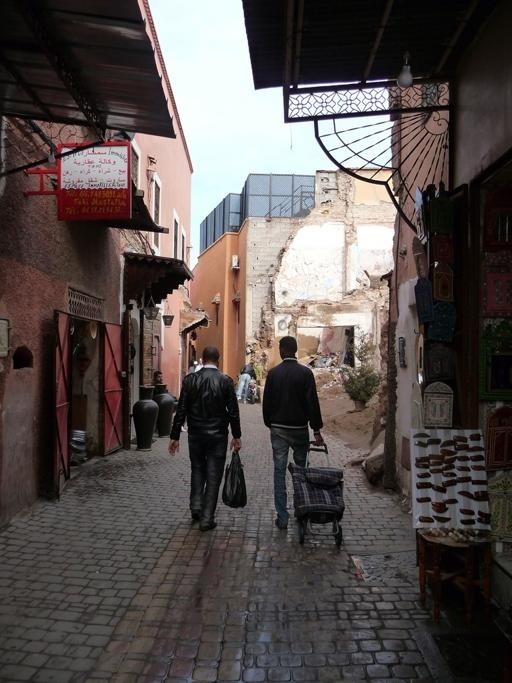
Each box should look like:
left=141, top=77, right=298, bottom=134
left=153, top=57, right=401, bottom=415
left=338, top=365, right=380, bottom=411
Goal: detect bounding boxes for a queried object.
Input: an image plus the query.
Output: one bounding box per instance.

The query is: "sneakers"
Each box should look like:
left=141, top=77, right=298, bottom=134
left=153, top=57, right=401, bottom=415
left=274, top=515, right=288, bottom=530
left=199, top=521, right=217, bottom=532
left=191, top=508, right=201, bottom=520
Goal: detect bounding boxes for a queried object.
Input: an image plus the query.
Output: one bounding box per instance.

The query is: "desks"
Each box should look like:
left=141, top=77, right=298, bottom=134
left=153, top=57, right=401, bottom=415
left=416, top=530, right=494, bottom=625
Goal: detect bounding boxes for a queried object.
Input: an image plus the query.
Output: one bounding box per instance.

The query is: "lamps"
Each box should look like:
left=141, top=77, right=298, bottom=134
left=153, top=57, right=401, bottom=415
left=396, top=52, right=414, bottom=89
left=143, top=294, right=160, bottom=320
left=47, top=120, right=56, bottom=163
left=163, top=303, right=174, bottom=326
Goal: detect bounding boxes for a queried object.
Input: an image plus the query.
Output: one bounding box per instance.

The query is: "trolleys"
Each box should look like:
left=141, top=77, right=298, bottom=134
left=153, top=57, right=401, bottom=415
left=290, top=438, right=346, bottom=546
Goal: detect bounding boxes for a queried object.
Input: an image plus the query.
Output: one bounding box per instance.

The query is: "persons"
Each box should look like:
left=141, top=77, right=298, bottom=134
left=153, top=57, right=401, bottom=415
left=168, top=346, right=241, bottom=531
left=262, top=336, right=324, bottom=528
left=195, top=357, right=203, bottom=372
left=236, top=361, right=256, bottom=404
left=151, top=371, right=187, bottom=432
left=189, top=361, right=199, bottom=373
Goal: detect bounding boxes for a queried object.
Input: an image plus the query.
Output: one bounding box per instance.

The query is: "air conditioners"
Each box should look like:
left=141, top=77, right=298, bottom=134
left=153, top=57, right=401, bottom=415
left=231, top=254, right=239, bottom=269
